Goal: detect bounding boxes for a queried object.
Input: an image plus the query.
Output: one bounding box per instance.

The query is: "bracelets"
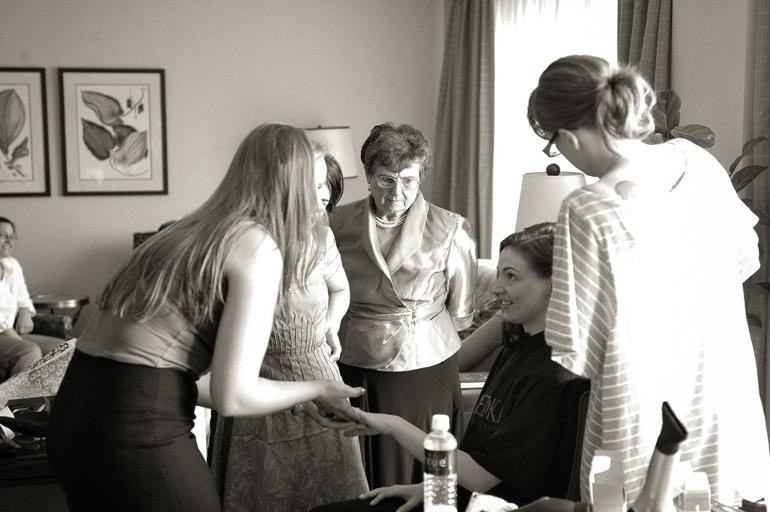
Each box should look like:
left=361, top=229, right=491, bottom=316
left=291, top=402, right=305, bottom=416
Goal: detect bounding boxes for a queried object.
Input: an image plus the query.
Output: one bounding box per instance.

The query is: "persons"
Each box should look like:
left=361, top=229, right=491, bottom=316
left=317, top=220, right=596, bottom=512
left=526, top=56, right=770, bottom=511
left=40, top=121, right=370, bottom=510
left=206, top=152, right=374, bottom=512
left=323, top=120, right=476, bottom=490
left=0, top=215, right=69, bottom=382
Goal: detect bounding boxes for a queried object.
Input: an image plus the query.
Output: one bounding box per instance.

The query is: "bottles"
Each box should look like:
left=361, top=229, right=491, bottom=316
left=422, top=412, right=459, bottom=511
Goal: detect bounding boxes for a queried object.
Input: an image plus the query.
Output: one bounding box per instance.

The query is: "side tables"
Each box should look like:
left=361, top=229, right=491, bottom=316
left=33, top=294, right=89, bottom=325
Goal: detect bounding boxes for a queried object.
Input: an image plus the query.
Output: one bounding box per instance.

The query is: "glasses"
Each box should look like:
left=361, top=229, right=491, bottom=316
left=542, top=126, right=578, bottom=158
left=368, top=169, right=420, bottom=189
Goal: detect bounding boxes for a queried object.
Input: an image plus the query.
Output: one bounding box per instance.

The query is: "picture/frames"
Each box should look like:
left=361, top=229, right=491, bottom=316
left=1, top=66, right=168, bottom=196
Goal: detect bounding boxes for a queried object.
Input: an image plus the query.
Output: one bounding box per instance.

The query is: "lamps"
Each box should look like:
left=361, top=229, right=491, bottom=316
left=305, top=125, right=359, bottom=180
left=515, top=163, right=587, bottom=233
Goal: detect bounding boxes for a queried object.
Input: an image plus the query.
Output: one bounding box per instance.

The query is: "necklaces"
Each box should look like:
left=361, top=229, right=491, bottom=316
left=376, top=215, right=406, bottom=228
left=0, top=263, right=4, bottom=281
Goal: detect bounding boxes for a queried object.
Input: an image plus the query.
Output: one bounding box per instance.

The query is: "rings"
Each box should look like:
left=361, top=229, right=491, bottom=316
left=356, top=407, right=360, bottom=415
